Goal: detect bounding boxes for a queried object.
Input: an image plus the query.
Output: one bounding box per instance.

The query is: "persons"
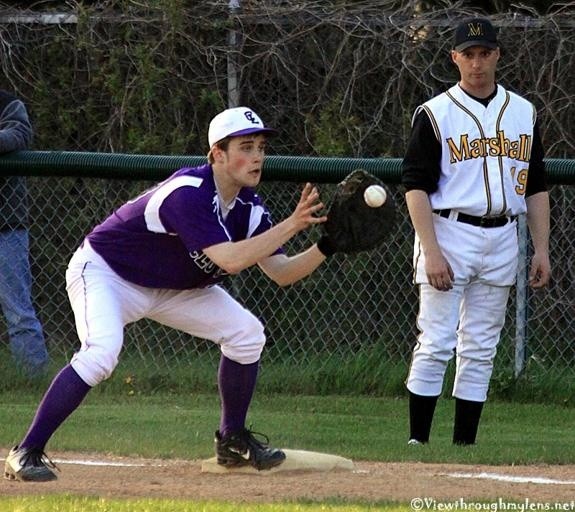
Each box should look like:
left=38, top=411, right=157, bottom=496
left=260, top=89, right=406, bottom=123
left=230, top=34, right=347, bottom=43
left=0, top=106, right=398, bottom=485
left=0, top=90, right=53, bottom=381
left=398, top=14, right=555, bottom=450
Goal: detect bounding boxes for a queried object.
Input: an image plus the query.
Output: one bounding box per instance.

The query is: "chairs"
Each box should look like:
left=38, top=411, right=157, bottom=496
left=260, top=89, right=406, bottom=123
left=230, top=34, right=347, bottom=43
left=213, top=427, right=287, bottom=473
left=404, top=438, right=430, bottom=451
left=3, top=445, right=59, bottom=486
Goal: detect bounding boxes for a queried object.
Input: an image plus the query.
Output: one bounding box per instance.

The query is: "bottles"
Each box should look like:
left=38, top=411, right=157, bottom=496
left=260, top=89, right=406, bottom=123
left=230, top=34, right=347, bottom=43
left=203, top=105, right=280, bottom=162
left=451, top=18, right=499, bottom=54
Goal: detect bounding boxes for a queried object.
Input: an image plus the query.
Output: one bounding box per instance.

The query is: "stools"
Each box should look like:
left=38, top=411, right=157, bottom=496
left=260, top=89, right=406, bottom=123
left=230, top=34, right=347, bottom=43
left=432, top=199, right=522, bottom=228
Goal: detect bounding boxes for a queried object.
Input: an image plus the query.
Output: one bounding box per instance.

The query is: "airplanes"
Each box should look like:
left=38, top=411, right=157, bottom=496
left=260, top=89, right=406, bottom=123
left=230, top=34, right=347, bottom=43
left=317, top=169, right=399, bottom=257
